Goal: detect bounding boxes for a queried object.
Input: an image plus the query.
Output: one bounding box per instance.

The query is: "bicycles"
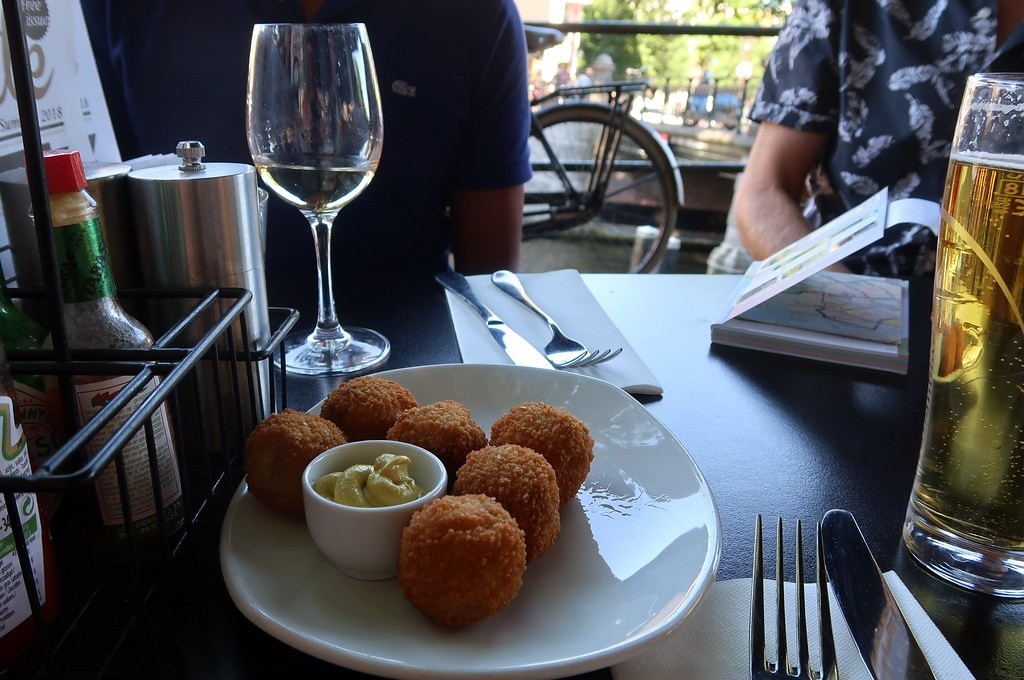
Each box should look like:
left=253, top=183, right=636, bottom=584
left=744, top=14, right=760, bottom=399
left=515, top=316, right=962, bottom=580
left=523, top=24, right=689, bottom=276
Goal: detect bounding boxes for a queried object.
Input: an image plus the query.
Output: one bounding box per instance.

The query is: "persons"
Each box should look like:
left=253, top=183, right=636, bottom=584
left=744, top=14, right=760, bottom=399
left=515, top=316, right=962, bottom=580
left=79, top=0, right=533, bottom=306
left=736, top=0, right=1024, bottom=279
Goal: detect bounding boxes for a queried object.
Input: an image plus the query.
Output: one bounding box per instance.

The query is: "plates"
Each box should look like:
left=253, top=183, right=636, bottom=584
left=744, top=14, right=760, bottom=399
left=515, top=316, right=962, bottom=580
left=223, top=364, right=723, bottom=680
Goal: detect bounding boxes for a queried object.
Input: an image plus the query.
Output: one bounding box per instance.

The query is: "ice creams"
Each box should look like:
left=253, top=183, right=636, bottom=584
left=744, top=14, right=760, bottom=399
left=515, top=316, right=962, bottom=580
left=311, top=452, right=423, bottom=508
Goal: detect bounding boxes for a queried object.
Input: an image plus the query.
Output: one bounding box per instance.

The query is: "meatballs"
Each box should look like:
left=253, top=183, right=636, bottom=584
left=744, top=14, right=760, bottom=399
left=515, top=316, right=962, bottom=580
left=245, top=377, right=593, bottom=625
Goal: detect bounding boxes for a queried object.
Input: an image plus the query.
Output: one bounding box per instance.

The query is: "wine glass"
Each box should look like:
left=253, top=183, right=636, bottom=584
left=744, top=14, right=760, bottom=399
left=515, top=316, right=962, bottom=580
left=246, top=22, right=391, bottom=376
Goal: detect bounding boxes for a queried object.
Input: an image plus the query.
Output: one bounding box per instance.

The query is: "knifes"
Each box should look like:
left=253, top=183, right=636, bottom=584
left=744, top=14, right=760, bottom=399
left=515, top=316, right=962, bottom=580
left=822, top=509, right=936, bottom=680
left=436, top=271, right=555, bottom=369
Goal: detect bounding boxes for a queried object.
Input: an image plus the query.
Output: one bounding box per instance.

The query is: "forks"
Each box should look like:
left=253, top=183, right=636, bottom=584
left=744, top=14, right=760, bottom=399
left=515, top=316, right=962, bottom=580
left=749, top=514, right=840, bottom=680
left=493, top=270, right=622, bottom=367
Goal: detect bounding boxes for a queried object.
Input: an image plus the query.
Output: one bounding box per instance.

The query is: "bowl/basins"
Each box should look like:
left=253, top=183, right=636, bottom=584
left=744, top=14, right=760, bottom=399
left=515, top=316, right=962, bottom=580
left=302, top=440, right=448, bottom=580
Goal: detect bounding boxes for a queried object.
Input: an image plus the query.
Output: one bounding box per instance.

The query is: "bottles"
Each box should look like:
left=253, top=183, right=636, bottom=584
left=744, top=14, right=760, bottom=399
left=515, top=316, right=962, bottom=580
left=22, top=152, right=185, bottom=545
left=0, top=263, right=70, bottom=471
left=0, top=344, right=60, bottom=665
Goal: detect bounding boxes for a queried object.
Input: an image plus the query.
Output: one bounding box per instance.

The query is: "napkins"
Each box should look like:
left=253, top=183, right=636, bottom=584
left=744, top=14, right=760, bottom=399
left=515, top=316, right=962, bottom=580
left=607, top=569, right=979, bottom=680
left=441, top=268, right=664, bottom=395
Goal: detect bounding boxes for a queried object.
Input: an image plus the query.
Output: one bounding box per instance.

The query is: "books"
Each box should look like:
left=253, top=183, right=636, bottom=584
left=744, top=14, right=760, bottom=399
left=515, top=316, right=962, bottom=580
left=709, top=184, right=911, bottom=377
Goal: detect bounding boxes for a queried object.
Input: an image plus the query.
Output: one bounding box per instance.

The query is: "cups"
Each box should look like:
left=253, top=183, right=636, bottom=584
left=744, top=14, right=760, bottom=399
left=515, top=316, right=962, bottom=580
left=259, top=188, right=268, bottom=259
left=904, top=72, right=1024, bottom=599
